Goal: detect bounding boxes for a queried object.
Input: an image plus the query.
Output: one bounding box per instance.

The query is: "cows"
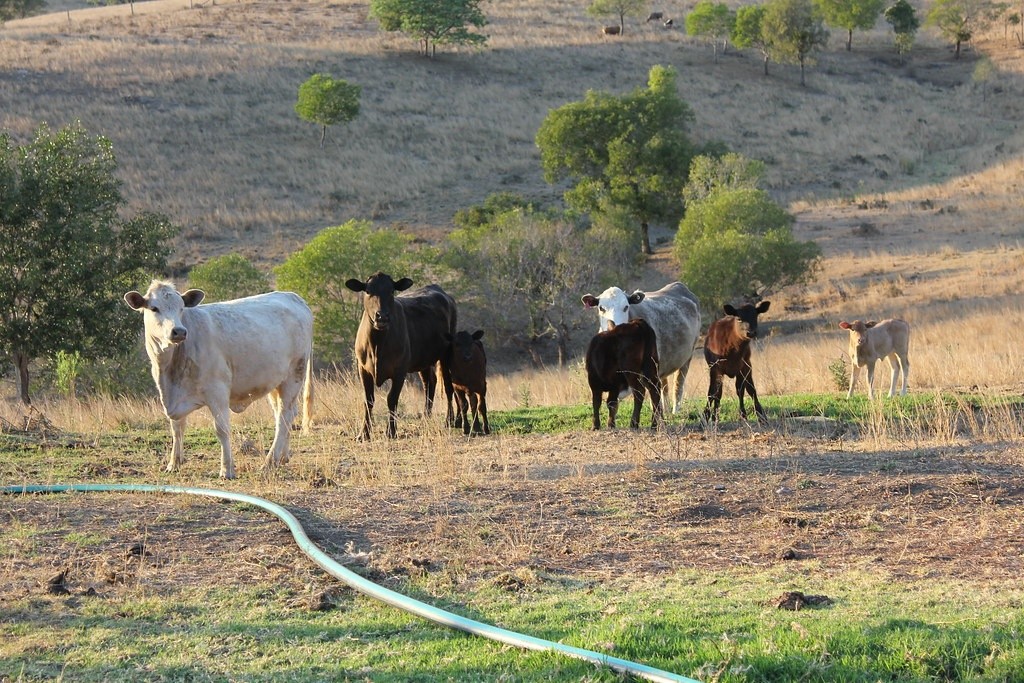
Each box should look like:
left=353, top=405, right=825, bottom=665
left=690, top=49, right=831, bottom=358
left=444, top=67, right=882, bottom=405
left=123, top=279, right=313, bottom=481
left=699, top=300, right=770, bottom=431
left=345, top=273, right=490, bottom=442
left=839, top=319, right=910, bottom=401
left=580, top=281, right=701, bottom=431
